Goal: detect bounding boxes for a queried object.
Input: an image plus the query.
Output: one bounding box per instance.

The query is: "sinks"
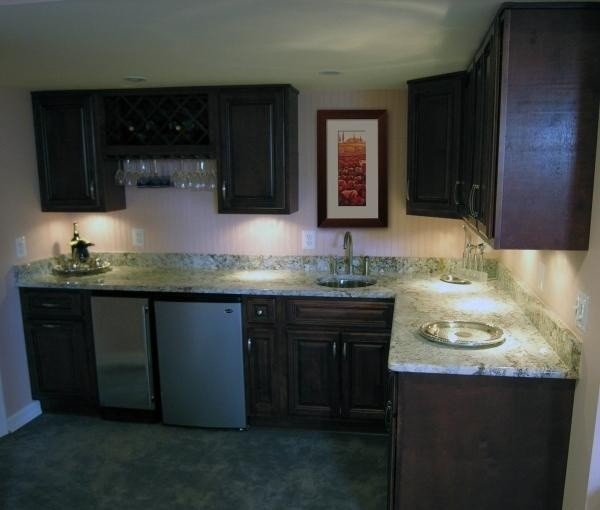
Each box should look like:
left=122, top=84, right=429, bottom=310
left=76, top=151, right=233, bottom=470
left=315, top=274, right=378, bottom=288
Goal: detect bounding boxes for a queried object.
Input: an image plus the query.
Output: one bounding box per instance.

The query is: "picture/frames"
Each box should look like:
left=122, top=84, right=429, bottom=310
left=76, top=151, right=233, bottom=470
left=316, top=109, right=387, bottom=226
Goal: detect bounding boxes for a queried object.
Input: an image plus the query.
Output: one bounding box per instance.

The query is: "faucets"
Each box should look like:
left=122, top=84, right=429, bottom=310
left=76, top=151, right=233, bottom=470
left=344, top=232, right=355, bottom=274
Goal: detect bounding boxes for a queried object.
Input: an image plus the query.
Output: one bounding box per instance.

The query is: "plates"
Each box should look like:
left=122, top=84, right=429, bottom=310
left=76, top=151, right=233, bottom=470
left=419, top=319, right=505, bottom=347
left=54, top=258, right=111, bottom=273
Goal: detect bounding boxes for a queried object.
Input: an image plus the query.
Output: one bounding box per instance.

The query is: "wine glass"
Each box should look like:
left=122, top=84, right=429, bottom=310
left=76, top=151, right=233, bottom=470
left=115, top=159, right=217, bottom=193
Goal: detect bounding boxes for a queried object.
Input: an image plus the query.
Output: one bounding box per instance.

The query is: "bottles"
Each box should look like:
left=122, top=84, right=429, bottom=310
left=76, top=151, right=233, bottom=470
left=474, top=243, right=488, bottom=281
left=463, top=244, right=474, bottom=281
left=71, top=222, right=80, bottom=257
left=440, top=275, right=473, bottom=284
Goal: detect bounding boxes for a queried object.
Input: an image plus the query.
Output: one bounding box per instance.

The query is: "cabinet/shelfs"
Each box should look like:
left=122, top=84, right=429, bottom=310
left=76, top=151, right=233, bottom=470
left=30, top=83, right=299, bottom=215
left=384, top=370, right=576, bottom=510
left=244, top=298, right=393, bottom=435
left=19, top=288, right=95, bottom=414
